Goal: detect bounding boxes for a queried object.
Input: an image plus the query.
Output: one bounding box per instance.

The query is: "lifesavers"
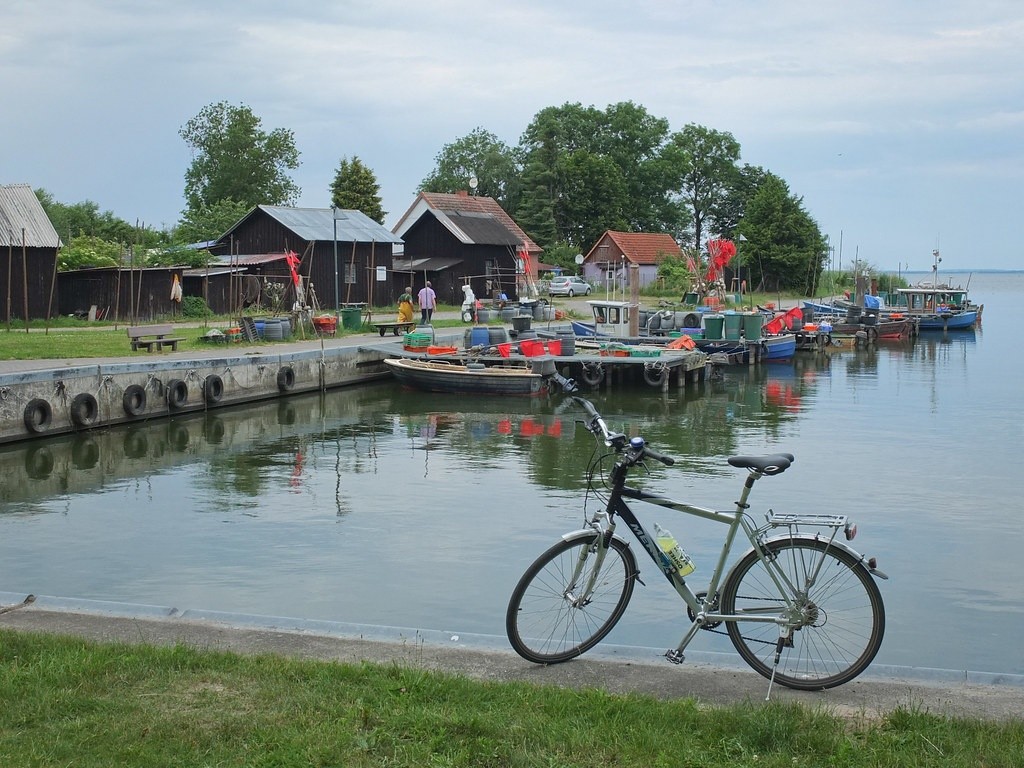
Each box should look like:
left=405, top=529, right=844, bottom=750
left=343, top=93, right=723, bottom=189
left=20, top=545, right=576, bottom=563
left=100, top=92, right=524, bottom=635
left=276, top=366, right=296, bottom=390
left=124, top=384, right=147, bottom=417
left=582, top=364, right=604, bottom=385
left=684, top=313, right=699, bottom=329
left=203, top=375, right=225, bottom=404
left=865, top=328, right=880, bottom=342
left=816, top=334, right=832, bottom=347
left=25, top=402, right=297, bottom=479
left=794, top=332, right=805, bottom=349
left=759, top=344, right=769, bottom=358
left=165, top=378, right=189, bottom=407
left=23, top=399, right=52, bottom=434
left=644, top=364, right=667, bottom=386
left=72, top=393, right=98, bottom=426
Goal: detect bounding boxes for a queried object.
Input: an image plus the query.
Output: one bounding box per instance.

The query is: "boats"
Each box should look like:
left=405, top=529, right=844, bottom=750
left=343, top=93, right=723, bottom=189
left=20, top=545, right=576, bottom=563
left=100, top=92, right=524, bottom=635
left=892, top=237, right=977, bottom=330
left=383, top=357, right=542, bottom=397
left=770, top=230, right=921, bottom=352
left=975, top=304, right=984, bottom=322
left=570, top=298, right=796, bottom=367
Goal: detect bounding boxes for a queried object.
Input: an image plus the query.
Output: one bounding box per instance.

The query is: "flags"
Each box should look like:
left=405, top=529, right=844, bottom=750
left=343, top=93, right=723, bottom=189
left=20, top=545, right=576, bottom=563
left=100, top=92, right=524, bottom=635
left=497, top=339, right=561, bottom=357
left=285, top=250, right=301, bottom=286
left=688, top=238, right=736, bottom=282
left=767, top=306, right=802, bottom=333
left=473, top=296, right=483, bottom=325
left=498, top=419, right=560, bottom=436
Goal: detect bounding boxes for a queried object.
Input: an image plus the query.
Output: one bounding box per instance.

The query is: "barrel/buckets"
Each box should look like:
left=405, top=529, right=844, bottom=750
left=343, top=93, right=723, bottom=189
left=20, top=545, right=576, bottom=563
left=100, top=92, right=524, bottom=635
left=793, top=308, right=814, bottom=331
left=415, top=325, right=434, bottom=345
left=498, top=340, right=562, bottom=357
left=518, top=330, right=537, bottom=355
left=512, top=317, right=532, bottom=329
left=724, top=314, right=763, bottom=339
left=556, top=330, right=575, bottom=356
left=648, top=312, right=660, bottom=328
left=847, top=307, right=880, bottom=324
left=703, top=317, right=724, bottom=339
left=471, top=327, right=489, bottom=346
left=253, top=315, right=292, bottom=341
left=639, top=310, right=647, bottom=326
left=488, top=327, right=508, bottom=344
left=477, top=305, right=555, bottom=324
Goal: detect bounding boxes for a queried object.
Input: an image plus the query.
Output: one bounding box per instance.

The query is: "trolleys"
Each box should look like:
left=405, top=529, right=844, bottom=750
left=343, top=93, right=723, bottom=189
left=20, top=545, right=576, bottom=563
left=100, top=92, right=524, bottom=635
left=369, top=305, right=420, bottom=336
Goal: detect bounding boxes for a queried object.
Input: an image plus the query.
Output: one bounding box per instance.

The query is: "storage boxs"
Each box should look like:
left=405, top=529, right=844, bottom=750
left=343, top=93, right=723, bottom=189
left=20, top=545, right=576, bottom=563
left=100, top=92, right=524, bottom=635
left=669, top=312, right=705, bottom=339
left=600, top=344, right=661, bottom=357
left=937, top=300, right=957, bottom=313
left=891, top=312, right=903, bottom=318
left=803, top=326, right=833, bottom=331
left=403, top=334, right=431, bottom=352
left=313, top=317, right=337, bottom=338
left=704, top=297, right=720, bottom=310
left=201, top=329, right=243, bottom=343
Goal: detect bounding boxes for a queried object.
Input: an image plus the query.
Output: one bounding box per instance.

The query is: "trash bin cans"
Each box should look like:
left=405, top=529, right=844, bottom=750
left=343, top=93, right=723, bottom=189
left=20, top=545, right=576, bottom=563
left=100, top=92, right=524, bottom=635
left=685, top=292, right=697, bottom=303
left=724, top=312, right=742, bottom=341
left=743, top=312, right=762, bottom=339
left=341, top=308, right=361, bottom=329
left=704, top=317, right=725, bottom=340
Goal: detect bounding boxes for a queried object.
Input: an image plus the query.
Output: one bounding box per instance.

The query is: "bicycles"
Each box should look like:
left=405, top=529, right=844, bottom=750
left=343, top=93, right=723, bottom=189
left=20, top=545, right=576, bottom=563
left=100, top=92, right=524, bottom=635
left=504, top=357, right=886, bottom=703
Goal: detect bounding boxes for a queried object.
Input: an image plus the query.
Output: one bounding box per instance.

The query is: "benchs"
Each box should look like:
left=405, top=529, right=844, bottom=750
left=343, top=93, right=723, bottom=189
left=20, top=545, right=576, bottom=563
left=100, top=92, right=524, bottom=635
left=127, top=325, right=187, bottom=353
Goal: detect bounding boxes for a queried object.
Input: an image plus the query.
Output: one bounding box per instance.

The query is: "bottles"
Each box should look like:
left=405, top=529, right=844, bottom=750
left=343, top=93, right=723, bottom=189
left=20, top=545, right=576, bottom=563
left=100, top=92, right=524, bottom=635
left=652, top=520, right=696, bottom=578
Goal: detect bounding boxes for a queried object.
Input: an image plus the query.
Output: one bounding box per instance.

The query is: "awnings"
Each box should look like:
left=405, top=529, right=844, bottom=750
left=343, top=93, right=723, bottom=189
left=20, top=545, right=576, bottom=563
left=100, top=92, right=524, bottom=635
left=392, top=256, right=464, bottom=272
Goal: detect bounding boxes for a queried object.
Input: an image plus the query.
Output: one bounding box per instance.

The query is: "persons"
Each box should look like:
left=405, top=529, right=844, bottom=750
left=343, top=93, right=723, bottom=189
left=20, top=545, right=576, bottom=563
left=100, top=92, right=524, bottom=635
left=498, top=290, right=508, bottom=316
left=397, top=287, right=415, bottom=336
left=418, top=281, right=436, bottom=325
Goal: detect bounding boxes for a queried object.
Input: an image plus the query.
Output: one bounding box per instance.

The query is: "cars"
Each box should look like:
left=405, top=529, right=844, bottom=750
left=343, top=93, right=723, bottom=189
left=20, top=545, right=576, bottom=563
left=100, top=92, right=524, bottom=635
left=549, top=275, right=592, bottom=298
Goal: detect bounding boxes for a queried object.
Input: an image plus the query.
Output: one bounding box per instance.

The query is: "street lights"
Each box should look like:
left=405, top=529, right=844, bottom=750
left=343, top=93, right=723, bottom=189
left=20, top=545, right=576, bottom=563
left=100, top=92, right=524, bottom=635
left=329, top=203, right=349, bottom=330
